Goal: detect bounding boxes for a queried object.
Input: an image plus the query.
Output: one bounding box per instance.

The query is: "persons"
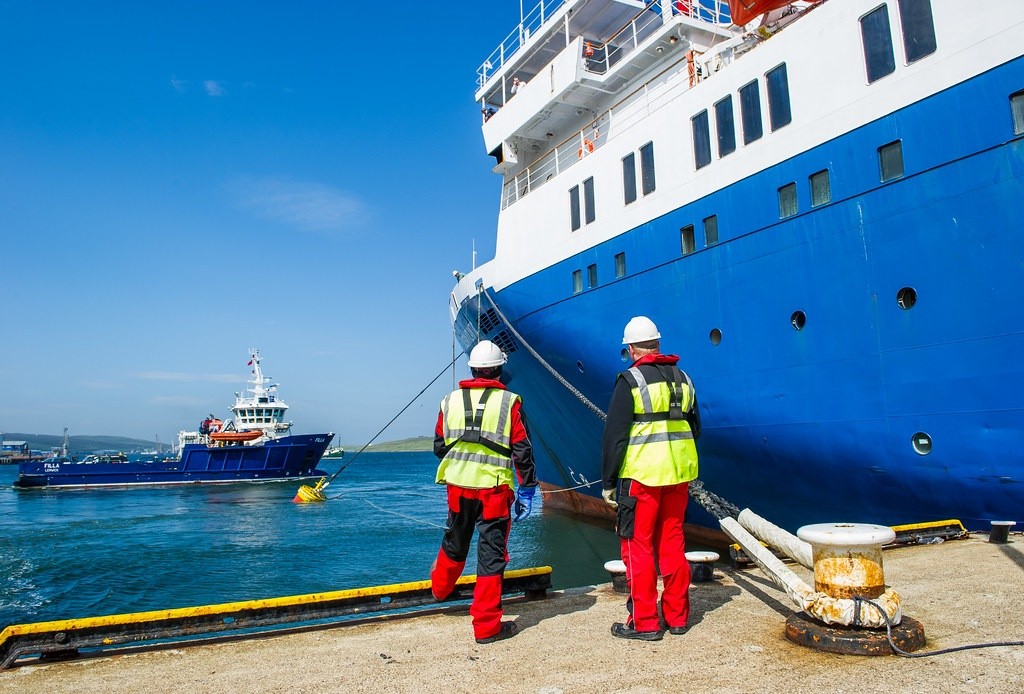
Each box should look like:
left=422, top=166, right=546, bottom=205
left=511, top=77, right=526, bottom=94
left=481, top=108, right=496, bottom=122
left=430, top=339, right=539, bottom=644
left=601, top=316, right=700, bottom=641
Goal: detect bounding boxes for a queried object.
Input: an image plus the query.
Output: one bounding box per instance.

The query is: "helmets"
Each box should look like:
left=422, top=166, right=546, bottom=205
left=467, top=339, right=508, bottom=368
left=622, top=315, right=661, bottom=345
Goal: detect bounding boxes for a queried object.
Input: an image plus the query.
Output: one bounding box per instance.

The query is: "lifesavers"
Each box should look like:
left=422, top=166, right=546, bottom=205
left=577, top=138, right=596, bottom=161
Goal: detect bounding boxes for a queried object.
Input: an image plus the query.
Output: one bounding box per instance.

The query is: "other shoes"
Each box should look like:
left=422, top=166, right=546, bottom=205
left=669, top=626, right=688, bottom=634
left=611, top=618, right=663, bottom=640
left=430, top=556, right=449, bottom=600
left=476, top=620, right=520, bottom=643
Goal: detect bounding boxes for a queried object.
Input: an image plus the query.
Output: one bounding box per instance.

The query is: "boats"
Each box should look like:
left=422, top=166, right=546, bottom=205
left=145, top=457, right=164, bottom=464
left=77, top=452, right=130, bottom=464
left=0, top=428, right=72, bottom=465
left=131, top=456, right=159, bottom=463
left=321, top=447, right=345, bottom=460
left=449, top=0, right=1024, bottom=551
left=11, top=347, right=335, bottom=489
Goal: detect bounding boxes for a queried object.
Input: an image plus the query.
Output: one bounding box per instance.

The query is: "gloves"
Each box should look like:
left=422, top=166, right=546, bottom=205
left=514, top=485, right=536, bottom=521
left=602, top=487, right=618, bottom=508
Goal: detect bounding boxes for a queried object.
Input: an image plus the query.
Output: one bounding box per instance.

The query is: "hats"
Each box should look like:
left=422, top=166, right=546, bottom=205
left=72, top=455, right=75, bottom=459
left=118, top=452, right=122, bottom=455
